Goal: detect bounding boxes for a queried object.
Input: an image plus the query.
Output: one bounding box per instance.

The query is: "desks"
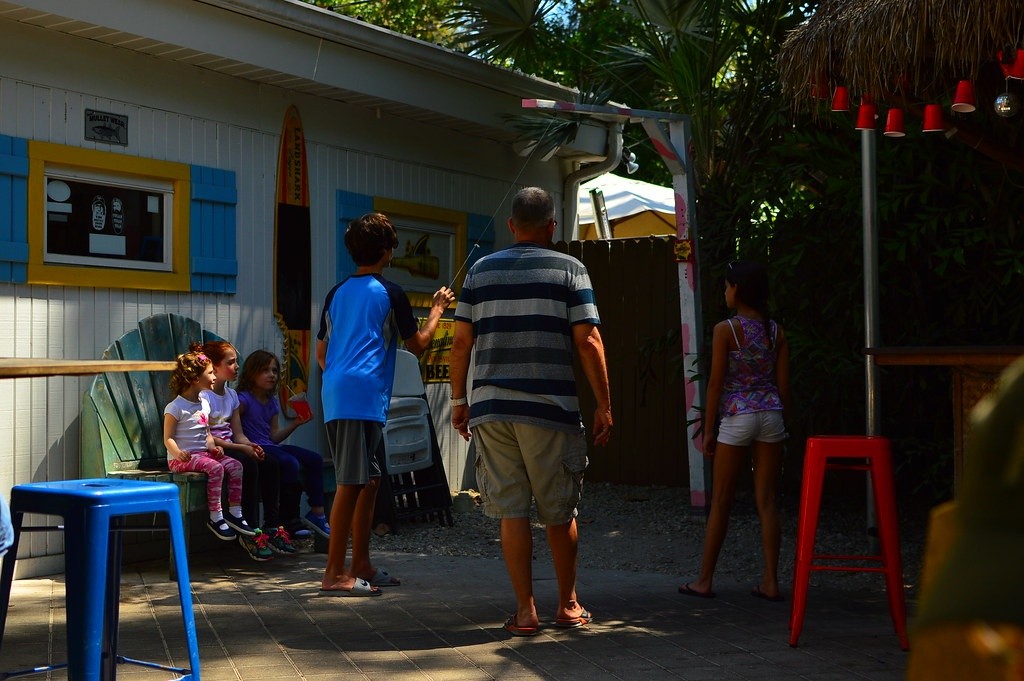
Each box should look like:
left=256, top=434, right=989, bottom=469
left=860, top=345, right=1024, bottom=503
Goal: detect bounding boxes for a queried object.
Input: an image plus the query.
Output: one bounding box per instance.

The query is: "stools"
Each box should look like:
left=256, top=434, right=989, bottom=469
left=787, top=436, right=913, bottom=651
left=0, top=478, right=202, bottom=681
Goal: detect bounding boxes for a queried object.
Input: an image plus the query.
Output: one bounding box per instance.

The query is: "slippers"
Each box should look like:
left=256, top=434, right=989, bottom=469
left=556, top=606, right=593, bottom=628
left=678, top=580, right=716, bottom=598
left=503, top=612, right=537, bottom=636
left=751, top=586, right=780, bottom=601
left=362, top=567, right=400, bottom=586
left=319, top=577, right=383, bottom=596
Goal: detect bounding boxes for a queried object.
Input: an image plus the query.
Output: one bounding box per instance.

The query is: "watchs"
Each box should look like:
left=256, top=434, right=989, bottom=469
left=448, top=396, right=468, bottom=406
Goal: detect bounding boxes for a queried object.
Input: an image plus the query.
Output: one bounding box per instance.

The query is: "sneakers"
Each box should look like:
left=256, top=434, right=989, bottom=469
left=260, top=526, right=296, bottom=555
left=239, top=527, right=274, bottom=561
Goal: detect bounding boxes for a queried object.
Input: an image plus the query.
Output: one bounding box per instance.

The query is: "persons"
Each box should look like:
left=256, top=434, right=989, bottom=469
left=449, top=186, right=613, bottom=635
left=315, top=211, right=456, bottom=597
left=235, top=350, right=332, bottom=538
left=189, top=340, right=297, bottom=561
left=163, top=350, right=256, bottom=540
left=678, top=258, right=790, bottom=602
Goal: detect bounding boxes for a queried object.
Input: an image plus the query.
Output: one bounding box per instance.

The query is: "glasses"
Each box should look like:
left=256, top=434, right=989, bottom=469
left=549, top=220, right=557, bottom=227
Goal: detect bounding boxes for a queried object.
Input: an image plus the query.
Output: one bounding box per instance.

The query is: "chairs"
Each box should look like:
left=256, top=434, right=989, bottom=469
left=327, top=349, right=454, bottom=536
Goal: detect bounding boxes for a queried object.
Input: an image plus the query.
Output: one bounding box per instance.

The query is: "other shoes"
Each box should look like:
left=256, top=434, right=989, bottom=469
left=224, top=513, right=256, bottom=536
left=206, top=518, right=236, bottom=541
left=288, top=518, right=312, bottom=541
left=304, top=510, right=331, bottom=538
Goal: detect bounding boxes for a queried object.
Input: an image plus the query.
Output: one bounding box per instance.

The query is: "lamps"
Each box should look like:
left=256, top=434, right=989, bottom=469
left=951, top=80, right=977, bottom=112
left=855, top=104, right=877, bottom=131
left=621, top=152, right=639, bottom=174
left=884, top=108, right=906, bottom=137
left=831, top=85, right=850, bottom=112
left=922, top=103, right=945, bottom=133
left=812, top=79, right=829, bottom=100
left=623, top=146, right=637, bottom=163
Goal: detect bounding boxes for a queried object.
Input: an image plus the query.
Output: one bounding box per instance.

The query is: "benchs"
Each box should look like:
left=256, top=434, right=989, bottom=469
left=78, top=313, right=260, bottom=580
